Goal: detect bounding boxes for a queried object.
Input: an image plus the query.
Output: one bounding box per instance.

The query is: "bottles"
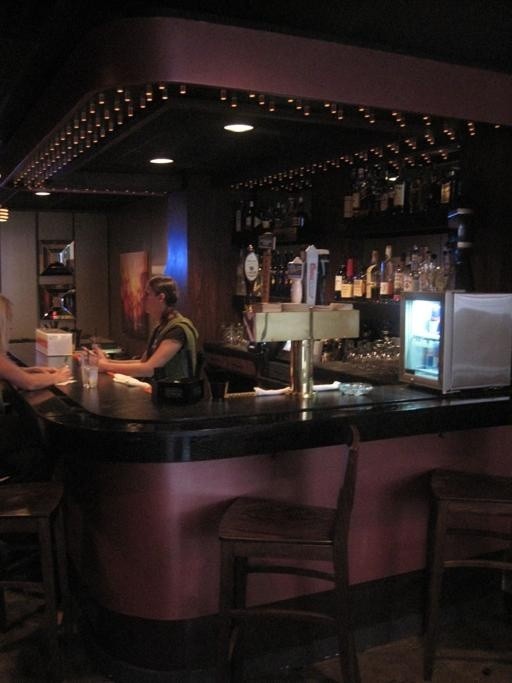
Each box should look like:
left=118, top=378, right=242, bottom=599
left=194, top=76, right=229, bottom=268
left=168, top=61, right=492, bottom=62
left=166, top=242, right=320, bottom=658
left=334, top=244, right=451, bottom=302
left=336, top=157, right=455, bottom=224
left=235, top=191, right=311, bottom=300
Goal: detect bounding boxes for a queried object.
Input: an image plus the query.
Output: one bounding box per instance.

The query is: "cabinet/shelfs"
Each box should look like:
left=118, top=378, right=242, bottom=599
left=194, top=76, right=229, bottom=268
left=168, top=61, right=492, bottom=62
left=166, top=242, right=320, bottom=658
left=233, top=157, right=466, bottom=375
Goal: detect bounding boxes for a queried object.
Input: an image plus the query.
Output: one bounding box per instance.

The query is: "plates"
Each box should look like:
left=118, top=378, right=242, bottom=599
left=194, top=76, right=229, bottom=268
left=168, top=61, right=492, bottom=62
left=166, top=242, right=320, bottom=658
left=338, top=383, right=373, bottom=395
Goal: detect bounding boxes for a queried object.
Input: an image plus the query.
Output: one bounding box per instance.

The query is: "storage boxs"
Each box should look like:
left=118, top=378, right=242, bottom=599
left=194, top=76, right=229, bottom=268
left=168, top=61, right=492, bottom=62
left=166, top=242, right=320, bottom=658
left=35, top=328, right=73, bottom=357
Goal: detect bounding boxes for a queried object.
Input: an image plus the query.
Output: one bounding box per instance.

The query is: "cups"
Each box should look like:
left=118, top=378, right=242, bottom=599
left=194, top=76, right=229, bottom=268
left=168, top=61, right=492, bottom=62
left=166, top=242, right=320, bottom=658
left=80, top=357, right=99, bottom=388
left=342, top=336, right=400, bottom=373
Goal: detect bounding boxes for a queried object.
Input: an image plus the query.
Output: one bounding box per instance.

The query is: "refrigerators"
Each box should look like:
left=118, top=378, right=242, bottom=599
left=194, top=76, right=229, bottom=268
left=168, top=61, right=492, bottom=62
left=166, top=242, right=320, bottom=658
left=395, top=288, right=512, bottom=395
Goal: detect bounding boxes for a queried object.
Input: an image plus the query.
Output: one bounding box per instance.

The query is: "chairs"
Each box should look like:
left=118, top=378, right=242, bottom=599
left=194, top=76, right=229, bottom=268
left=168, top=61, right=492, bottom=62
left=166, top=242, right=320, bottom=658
left=215, top=423, right=364, bottom=683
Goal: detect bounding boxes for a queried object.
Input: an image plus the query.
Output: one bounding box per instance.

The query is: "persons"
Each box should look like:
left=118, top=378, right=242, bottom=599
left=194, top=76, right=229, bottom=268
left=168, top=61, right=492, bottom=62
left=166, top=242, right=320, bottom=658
left=0, top=296, right=82, bottom=592
left=78, top=277, right=215, bottom=403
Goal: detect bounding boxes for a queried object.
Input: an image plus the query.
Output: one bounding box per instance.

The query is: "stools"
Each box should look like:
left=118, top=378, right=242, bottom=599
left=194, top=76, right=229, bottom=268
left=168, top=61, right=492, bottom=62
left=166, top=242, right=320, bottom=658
left=421, top=468, right=511, bottom=683
left=0, top=480, right=75, bottom=682
